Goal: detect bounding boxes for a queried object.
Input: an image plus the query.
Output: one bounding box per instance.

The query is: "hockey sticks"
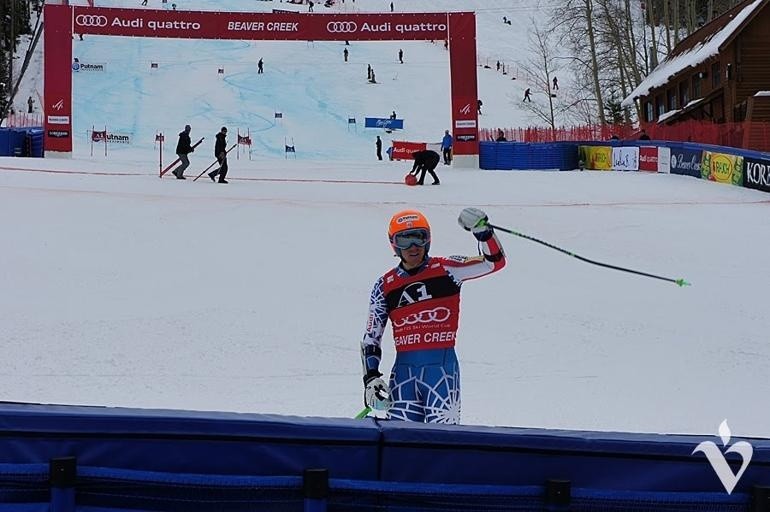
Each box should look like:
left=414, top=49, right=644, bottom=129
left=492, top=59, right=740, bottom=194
left=161, top=137, right=205, bottom=177
left=194, top=144, right=237, bottom=180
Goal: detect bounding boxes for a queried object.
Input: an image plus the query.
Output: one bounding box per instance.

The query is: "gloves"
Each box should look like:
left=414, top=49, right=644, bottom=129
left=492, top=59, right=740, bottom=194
left=360, top=374, right=393, bottom=408
left=457, top=207, right=494, bottom=241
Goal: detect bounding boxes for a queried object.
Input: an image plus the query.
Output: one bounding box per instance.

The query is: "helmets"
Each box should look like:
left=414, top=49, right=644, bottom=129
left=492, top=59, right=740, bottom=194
left=388, top=209, right=431, bottom=255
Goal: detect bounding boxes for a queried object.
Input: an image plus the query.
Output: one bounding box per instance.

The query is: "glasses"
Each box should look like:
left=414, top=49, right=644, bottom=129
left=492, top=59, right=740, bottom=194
left=388, top=227, right=431, bottom=250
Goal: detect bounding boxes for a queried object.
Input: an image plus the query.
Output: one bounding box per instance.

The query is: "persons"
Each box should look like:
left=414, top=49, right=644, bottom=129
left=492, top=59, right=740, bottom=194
left=496, top=59, right=500, bottom=71
left=257, top=56, right=266, bottom=75
left=607, top=132, right=620, bottom=140
left=639, top=129, right=650, bottom=141
left=478, top=99, right=482, bottom=115
left=390, top=2, right=394, bottom=12
left=552, top=76, right=559, bottom=91
left=410, top=149, right=441, bottom=186
left=444, top=39, right=449, bottom=50
left=371, top=69, right=376, bottom=82
left=170, top=123, right=197, bottom=180
left=345, top=41, right=350, bottom=45
left=367, top=64, right=371, bottom=79
left=360, top=207, right=507, bottom=427
left=306, top=0, right=315, bottom=13
left=344, top=48, right=349, bottom=62
left=398, top=49, right=404, bottom=65
left=441, top=129, right=454, bottom=166
left=27, top=96, right=36, bottom=113
left=390, top=111, right=396, bottom=121
left=522, top=88, right=533, bottom=103
left=375, top=135, right=383, bottom=161
left=496, top=131, right=507, bottom=142
left=208, top=127, right=229, bottom=184
left=172, top=4, right=176, bottom=11
left=142, top=0, right=147, bottom=6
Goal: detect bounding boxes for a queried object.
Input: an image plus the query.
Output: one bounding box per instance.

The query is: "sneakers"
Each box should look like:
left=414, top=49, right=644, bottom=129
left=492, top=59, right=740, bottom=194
left=218, top=179, right=228, bottom=183
left=207, top=173, right=215, bottom=182
